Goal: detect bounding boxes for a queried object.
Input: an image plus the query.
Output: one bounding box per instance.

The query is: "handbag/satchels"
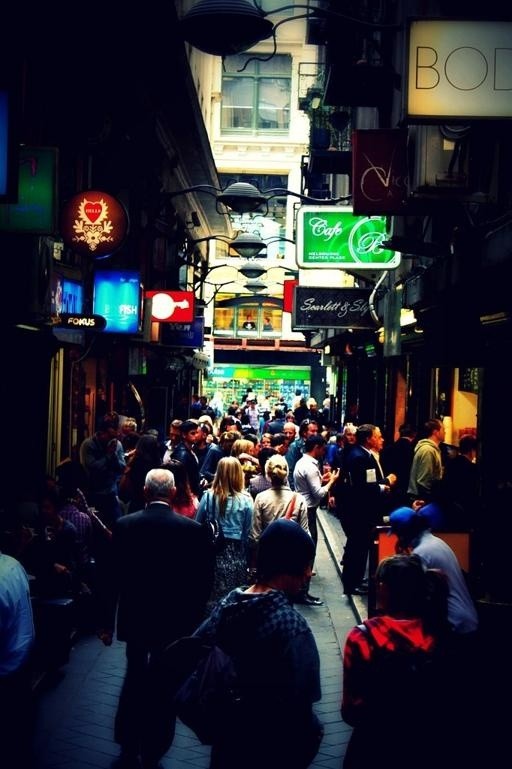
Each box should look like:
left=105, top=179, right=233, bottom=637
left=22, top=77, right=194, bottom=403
left=160, top=635, right=232, bottom=745
left=202, top=510, right=225, bottom=552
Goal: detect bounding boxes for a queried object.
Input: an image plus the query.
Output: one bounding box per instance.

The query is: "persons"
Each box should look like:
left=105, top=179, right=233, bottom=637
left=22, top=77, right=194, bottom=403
left=2, top=390, right=476, bottom=767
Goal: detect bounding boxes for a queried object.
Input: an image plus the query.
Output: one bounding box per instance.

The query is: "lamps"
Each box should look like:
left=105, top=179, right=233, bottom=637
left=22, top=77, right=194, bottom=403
left=169, top=179, right=352, bottom=310
left=183, top=0, right=403, bottom=92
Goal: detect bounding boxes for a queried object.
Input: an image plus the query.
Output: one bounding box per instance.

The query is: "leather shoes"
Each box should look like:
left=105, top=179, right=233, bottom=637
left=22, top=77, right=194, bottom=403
left=348, top=587, right=367, bottom=595
left=297, top=594, right=322, bottom=605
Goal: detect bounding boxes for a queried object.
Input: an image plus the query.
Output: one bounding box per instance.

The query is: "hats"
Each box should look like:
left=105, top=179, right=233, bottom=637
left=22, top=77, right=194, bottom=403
left=387, top=506, right=417, bottom=537
left=259, top=519, right=315, bottom=561
